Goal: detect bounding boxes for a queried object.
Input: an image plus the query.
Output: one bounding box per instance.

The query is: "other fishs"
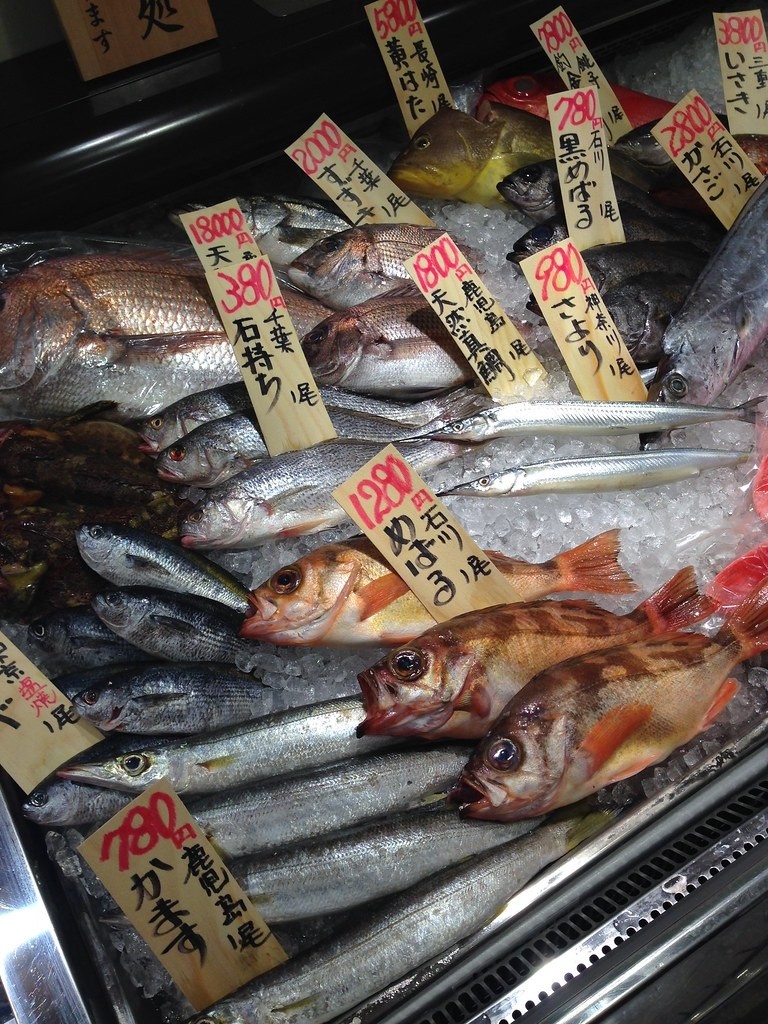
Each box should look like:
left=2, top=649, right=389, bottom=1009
left=0, top=86, right=768, bottom=1023
